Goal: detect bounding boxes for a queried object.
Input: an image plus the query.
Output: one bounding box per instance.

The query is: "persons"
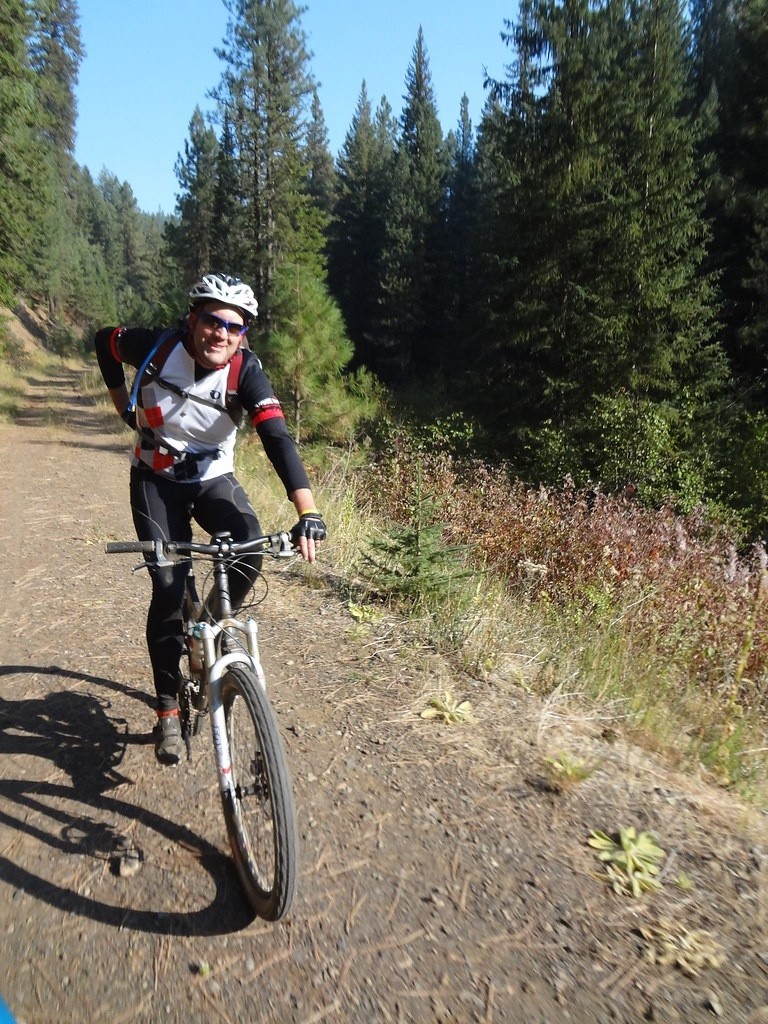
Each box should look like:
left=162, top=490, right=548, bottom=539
left=94, top=275, right=327, bottom=764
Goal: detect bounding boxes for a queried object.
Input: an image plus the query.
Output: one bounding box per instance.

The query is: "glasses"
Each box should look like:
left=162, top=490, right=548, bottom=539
left=198, top=312, right=246, bottom=335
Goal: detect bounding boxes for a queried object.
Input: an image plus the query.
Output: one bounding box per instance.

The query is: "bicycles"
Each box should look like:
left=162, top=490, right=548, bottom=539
left=103, top=500, right=303, bottom=923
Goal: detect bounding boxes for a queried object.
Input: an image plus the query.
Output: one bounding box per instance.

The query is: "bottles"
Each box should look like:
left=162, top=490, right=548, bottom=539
left=190, top=621, right=212, bottom=673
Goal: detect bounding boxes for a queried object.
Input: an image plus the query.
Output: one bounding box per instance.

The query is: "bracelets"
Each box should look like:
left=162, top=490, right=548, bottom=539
left=299, top=510, right=319, bottom=519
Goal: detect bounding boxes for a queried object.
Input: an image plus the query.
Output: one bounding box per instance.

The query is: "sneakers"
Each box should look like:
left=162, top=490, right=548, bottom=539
left=155, top=715, right=183, bottom=764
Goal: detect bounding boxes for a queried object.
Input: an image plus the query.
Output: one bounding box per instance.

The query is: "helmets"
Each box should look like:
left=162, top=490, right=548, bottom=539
left=189, top=273, right=259, bottom=316
